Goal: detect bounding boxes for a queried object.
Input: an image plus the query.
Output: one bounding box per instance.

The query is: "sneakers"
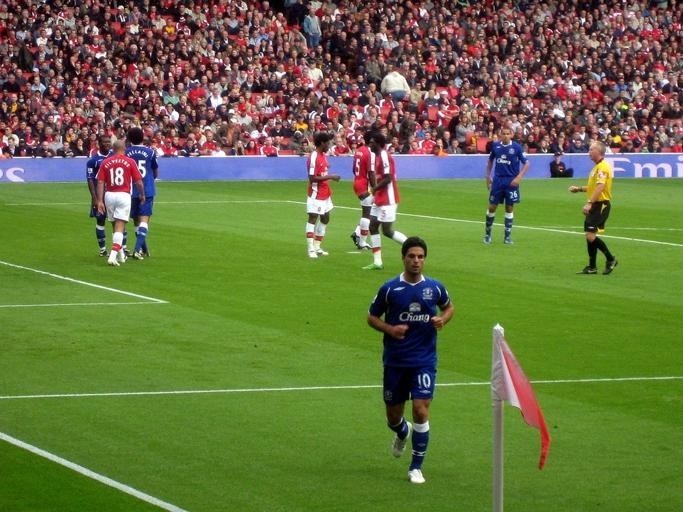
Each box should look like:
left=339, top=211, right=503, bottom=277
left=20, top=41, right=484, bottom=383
left=99, top=245, right=151, bottom=266
left=350, top=230, right=383, bottom=269
left=504, top=237, right=512, bottom=244
left=407, top=469, right=426, bottom=484
left=484, top=235, right=491, bottom=244
left=602, top=256, right=617, bottom=274
left=391, top=421, right=413, bottom=458
left=576, top=266, right=597, bottom=274
left=306, top=247, right=330, bottom=259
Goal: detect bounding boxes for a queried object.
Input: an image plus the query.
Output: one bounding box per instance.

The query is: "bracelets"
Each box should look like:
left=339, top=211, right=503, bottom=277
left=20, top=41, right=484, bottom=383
left=367, top=187, right=374, bottom=195
left=577, top=184, right=583, bottom=191
left=587, top=199, right=594, bottom=204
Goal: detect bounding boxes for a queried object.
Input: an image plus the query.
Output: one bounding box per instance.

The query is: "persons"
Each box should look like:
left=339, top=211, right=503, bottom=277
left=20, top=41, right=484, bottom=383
left=95, top=140, right=146, bottom=266
left=358, top=133, right=411, bottom=270
left=85, top=135, right=133, bottom=258
left=568, top=140, right=619, bottom=274
left=127, top=128, right=159, bottom=261
left=305, top=132, right=340, bottom=258
left=549, top=150, right=574, bottom=178
left=482, top=127, right=530, bottom=245
left=0, top=0, right=683, bottom=161
left=350, top=130, right=375, bottom=251
left=366, top=236, right=454, bottom=484
